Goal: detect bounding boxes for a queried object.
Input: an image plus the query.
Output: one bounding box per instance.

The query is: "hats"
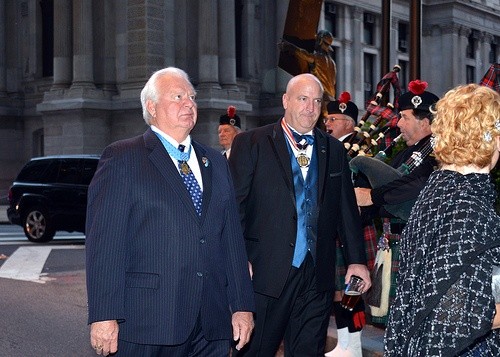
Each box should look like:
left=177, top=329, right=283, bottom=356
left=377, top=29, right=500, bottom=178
left=219, top=105, right=241, bottom=129
left=327, top=91, right=358, bottom=126
left=398, top=79, right=440, bottom=111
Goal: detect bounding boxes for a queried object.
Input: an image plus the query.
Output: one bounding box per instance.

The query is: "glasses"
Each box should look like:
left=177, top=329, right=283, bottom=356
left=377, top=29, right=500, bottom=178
left=322, top=117, right=349, bottom=123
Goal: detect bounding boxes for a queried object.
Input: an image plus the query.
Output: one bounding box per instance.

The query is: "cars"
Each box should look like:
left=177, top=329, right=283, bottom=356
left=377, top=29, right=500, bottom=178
left=6, top=154, right=101, bottom=241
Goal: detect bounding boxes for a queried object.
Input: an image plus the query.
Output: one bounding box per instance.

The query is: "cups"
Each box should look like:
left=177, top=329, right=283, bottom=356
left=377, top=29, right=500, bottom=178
left=340, top=275, right=366, bottom=312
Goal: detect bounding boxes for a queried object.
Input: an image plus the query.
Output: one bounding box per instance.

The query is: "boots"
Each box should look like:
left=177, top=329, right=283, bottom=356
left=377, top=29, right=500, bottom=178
left=324, top=326, right=348, bottom=357
left=337, top=330, right=362, bottom=356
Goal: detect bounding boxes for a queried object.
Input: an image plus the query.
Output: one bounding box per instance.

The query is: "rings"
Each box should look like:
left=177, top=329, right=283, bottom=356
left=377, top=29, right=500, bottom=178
left=95, top=343, right=103, bottom=350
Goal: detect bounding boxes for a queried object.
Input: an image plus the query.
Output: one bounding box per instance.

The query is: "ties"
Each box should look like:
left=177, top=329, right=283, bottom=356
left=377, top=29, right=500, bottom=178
left=177, top=144, right=203, bottom=219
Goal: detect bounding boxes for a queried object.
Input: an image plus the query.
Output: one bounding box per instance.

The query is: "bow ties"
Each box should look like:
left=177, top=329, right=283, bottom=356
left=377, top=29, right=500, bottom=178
left=287, top=124, right=315, bottom=145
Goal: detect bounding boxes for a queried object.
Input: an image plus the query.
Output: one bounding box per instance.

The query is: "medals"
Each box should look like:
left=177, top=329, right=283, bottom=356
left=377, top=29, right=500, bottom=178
left=297, top=152, right=310, bottom=169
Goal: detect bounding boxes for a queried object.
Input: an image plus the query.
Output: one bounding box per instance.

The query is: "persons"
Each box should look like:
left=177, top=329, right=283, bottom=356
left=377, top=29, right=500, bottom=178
left=87, top=66, right=255, bottom=357
left=228, top=73, right=372, bottom=357
left=277, top=28, right=336, bottom=116
left=218, top=106, right=242, bottom=158
left=323, top=79, right=441, bottom=333
left=384, top=83, right=500, bottom=357
